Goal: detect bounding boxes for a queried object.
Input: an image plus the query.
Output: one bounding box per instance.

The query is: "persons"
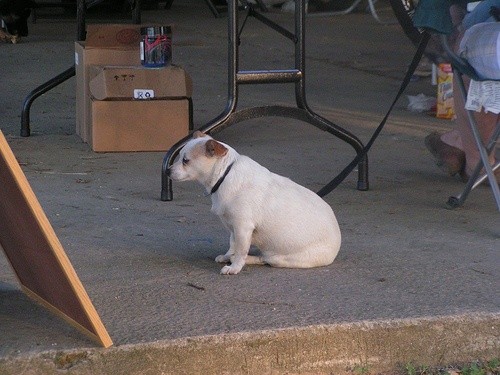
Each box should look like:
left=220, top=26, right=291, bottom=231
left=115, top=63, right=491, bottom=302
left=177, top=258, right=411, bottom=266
left=423, top=0, right=499, bottom=188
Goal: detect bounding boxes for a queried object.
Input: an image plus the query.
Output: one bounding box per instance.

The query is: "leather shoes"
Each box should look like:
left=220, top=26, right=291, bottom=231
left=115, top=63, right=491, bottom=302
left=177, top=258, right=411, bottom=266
left=423, top=129, right=464, bottom=175
left=459, top=159, right=500, bottom=185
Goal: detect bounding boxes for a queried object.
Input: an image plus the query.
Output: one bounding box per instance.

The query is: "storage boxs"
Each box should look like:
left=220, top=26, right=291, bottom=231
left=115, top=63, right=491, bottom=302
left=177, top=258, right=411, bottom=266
left=436, top=62, right=460, bottom=121
left=71, top=22, right=148, bottom=144
left=86, top=60, right=197, bottom=154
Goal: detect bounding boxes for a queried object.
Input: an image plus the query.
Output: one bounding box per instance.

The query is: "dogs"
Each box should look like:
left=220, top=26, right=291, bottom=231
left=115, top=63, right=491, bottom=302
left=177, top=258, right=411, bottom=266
left=165, top=130, right=342, bottom=275
